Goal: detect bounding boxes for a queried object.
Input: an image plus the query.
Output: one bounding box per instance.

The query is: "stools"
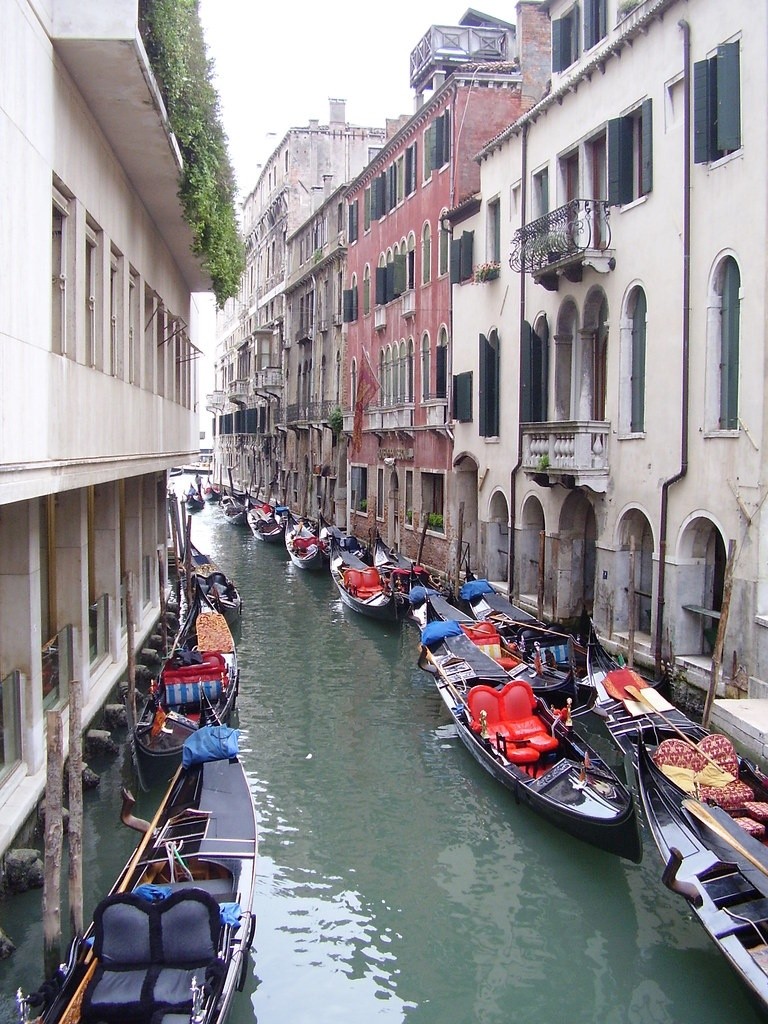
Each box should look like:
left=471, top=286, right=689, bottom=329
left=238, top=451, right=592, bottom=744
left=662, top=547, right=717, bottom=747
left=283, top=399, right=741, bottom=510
left=151, top=1007, right=192, bottom=1024
left=497, top=658, right=518, bottom=670
left=524, top=731, right=559, bottom=770
left=743, top=801, right=768, bottom=842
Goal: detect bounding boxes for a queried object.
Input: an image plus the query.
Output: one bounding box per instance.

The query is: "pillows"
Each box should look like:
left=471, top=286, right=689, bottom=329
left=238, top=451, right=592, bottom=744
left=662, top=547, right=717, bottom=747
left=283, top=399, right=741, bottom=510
left=209, top=582, right=227, bottom=596
left=200, top=584, right=208, bottom=594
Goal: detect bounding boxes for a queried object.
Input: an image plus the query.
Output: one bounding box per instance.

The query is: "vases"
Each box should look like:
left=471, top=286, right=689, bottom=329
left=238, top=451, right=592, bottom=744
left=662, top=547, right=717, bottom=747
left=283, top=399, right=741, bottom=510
left=314, top=466, right=321, bottom=474
left=480, top=270, right=499, bottom=281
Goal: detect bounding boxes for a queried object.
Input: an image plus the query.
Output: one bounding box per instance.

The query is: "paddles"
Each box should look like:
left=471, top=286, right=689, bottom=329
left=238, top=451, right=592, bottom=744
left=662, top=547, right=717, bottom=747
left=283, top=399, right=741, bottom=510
left=624, top=684, right=727, bottom=775
left=489, top=615, right=569, bottom=637
left=681, top=799, right=768, bottom=889
left=84, top=762, right=184, bottom=965
left=416, top=641, right=473, bottom=714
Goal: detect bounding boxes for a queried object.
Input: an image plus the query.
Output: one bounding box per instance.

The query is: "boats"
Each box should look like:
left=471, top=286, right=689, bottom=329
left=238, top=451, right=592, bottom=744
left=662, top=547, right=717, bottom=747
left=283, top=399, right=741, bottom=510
left=405, top=562, right=576, bottom=705
left=18, top=674, right=258, bottom=1024
left=243, top=489, right=286, bottom=543
left=274, top=499, right=319, bottom=535
left=187, top=483, right=205, bottom=510
left=183, top=526, right=243, bottom=616
left=633, top=722, right=768, bottom=1011
left=204, top=478, right=221, bottom=502
left=586, top=616, right=768, bottom=889
left=419, top=594, right=633, bottom=830
left=462, top=694, right=484, bottom=708
left=459, top=561, right=671, bottom=700
left=372, top=527, right=454, bottom=605
left=327, top=530, right=402, bottom=621
left=219, top=487, right=248, bottom=525
left=134, top=567, right=240, bottom=756
left=317, top=510, right=365, bottom=562
left=285, top=510, right=324, bottom=571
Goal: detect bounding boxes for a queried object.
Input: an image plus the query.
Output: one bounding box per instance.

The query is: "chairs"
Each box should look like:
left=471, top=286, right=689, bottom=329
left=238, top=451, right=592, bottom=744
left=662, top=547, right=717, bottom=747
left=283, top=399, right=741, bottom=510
left=706, top=796, right=766, bottom=842
left=653, top=739, right=709, bottom=805
left=344, top=536, right=358, bottom=553
left=354, top=584, right=372, bottom=599
left=470, top=684, right=511, bottom=742
left=209, top=572, right=226, bottom=591
left=459, top=623, right=473, bottom=638
left=697, top=734, right=754, bottom=816
left=151, top=887, right=223, bottom=1013
left=306, top=537, right=319, bottom=547
left=294, top=539, right=306, bottom=550
left=81, top=891, right=151, bottom=1024
left=502, top=679, right=549, bottom=747
left=362, top=568, right=384, bottom=593
left=191, top=575, right=208, bottom=594
left=343, top=569, right=365, bottom=592
left=495, top=732, right=542, bottom=779
left=474, top=622, right=496, bottom=637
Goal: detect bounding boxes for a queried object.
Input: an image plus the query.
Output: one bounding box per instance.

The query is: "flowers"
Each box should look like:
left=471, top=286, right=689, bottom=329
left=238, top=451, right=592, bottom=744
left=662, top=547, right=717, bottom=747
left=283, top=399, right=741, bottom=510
left=469, top=260, right=501, bottom=285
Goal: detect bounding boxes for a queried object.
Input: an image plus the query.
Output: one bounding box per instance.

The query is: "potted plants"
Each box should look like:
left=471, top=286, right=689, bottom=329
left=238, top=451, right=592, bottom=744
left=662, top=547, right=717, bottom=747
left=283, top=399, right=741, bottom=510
left=330, top=497, right=335, bottom=514
left=435, top=514, right=444, bottom=533
left=360, top=498, right=367, bottom=513
left=530, top=233, right=567, bottom=263
left=429, top=513, right=438, bottom=530
left=408, top=510, right=412, bottom=525
left=424, top=512, right=431, bottom=530
